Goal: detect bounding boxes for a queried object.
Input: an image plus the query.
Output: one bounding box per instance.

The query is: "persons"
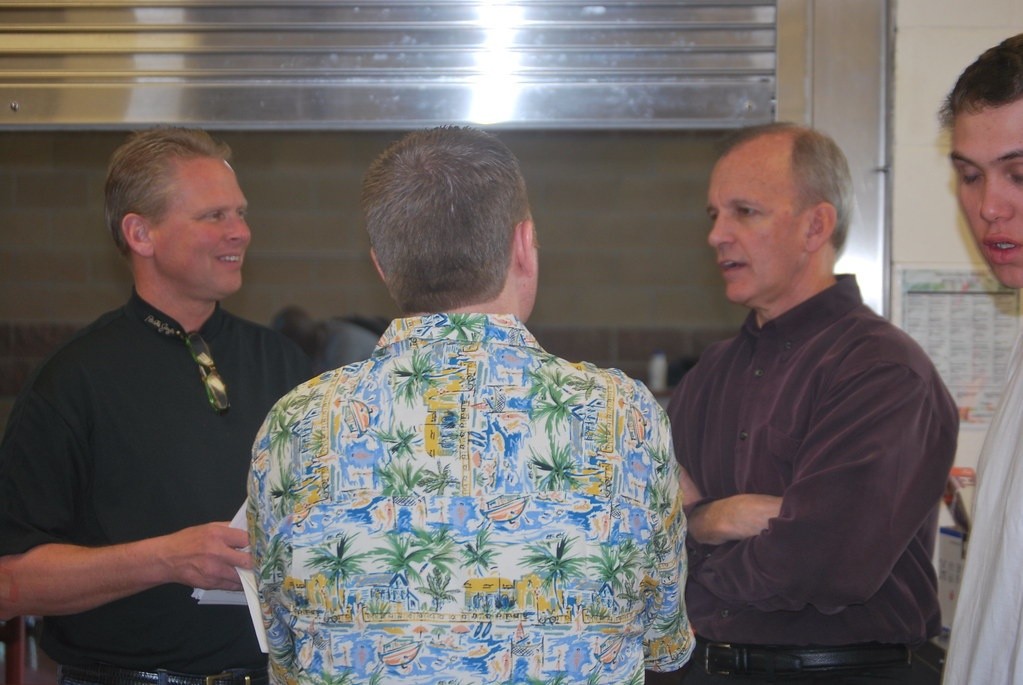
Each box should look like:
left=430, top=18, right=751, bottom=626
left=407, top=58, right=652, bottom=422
left=666, top=121, right=960, bottom=685
left=1, top=122, right=317, bottom=684
left=247, top=123, right=696, bottom=685
left=270, top=304, right=381, bottom=377
left=940, top=30, right=1023, bottom=685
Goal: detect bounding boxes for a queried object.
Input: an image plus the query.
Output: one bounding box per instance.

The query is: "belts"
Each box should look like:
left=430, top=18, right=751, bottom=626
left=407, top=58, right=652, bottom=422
left=691, top=634, right=908, bottom=676
left=56, top=661, right=269, bottom=685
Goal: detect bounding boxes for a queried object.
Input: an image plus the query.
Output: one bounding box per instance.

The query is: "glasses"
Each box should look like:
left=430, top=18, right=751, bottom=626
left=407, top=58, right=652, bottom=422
left=185, top=332, right=231, bottom=415
